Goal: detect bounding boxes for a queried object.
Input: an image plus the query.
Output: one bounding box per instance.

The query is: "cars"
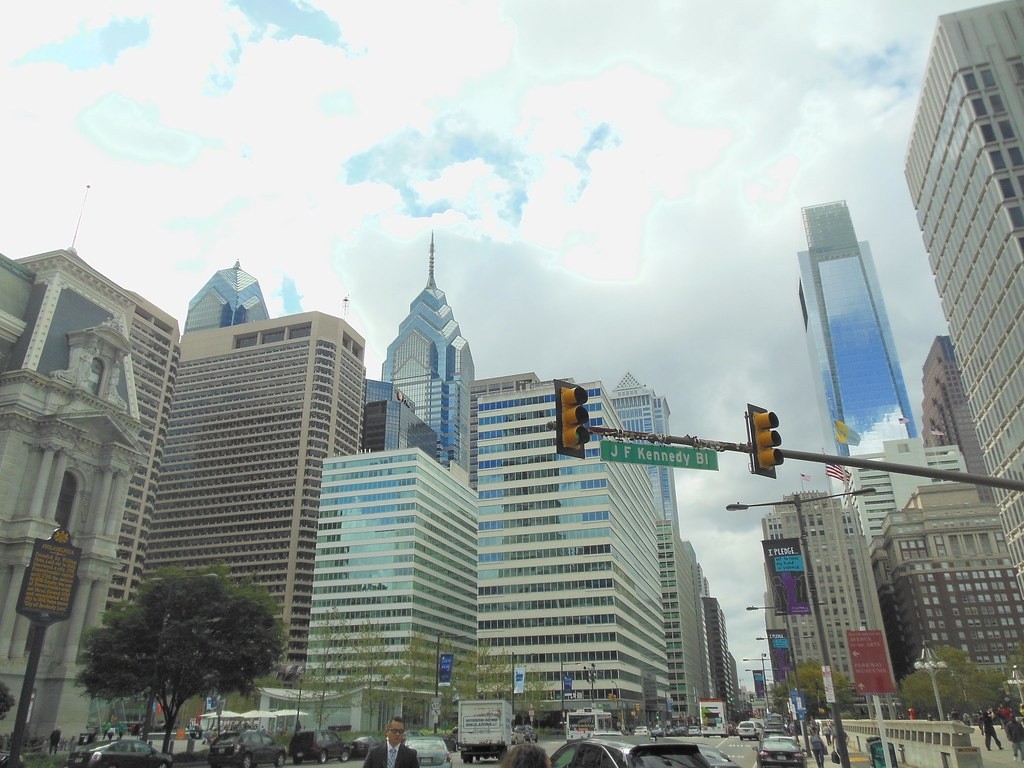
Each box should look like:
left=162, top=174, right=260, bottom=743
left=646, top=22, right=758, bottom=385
left=68, top=696, right=813, bottom=768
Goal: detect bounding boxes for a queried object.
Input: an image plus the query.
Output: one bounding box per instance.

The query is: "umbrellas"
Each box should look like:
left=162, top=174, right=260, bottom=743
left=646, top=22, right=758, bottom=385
left=199, top=709, right=308, bottom=733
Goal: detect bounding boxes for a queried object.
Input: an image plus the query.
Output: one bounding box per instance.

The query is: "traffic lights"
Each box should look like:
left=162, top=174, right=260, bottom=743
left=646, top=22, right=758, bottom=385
left=554, top=383, right=591, bottom=458
left=752, top=409, right=783, bottom=474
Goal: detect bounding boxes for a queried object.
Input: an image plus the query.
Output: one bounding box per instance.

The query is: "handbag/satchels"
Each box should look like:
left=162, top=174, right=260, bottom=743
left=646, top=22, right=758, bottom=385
left=822, top=745, right=828, bottom=755
left=831, top=748, right=840, bottom=764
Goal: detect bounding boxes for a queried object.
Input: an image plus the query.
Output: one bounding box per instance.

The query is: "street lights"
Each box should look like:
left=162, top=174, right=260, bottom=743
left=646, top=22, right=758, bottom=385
left=1007, top=664, right=1024, bottom=703
left=560, top=662, right=582, bottom=729
left=724, top=487, right=879, bottom=768
left=433, top=633, right=467, bottom=733
left=745, top=669, right=764, bottom=715
left=914, top=639, right=950, bottom=722
left=745, top=601, right=829, bottom=758
left=756, top=634, right=813, bottom=744
left=743, top=658, right=771, bottom=716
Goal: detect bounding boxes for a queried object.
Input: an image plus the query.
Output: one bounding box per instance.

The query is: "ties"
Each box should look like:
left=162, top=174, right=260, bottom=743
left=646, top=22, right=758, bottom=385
left=387, top=748, right=398, bottom=768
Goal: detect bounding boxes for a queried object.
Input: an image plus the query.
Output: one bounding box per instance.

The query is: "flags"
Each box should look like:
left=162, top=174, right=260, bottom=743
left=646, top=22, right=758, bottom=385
left=825, top=461, right=852, bottom=483
left=835, top=417, right=861, bottom=446
left=931, top=428, right=944, bottom=435
left=801, top=473, right=811, bottom=481
left=899, top=418, right=910, bottom=424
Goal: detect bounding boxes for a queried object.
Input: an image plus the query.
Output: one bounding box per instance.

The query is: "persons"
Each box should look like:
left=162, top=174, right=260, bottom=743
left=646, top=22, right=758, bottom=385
left=787, top=720, right=850, bottom=768
left=362, top=716, right=420, bottom=768
left=897, top=703, right=1024, bottom=764
left=103, top=721, right=144, bottom=740
left=48, top=726, right=62, bottom=755
left=626, top=724, right=677, bottom=737
left=202, top=721, right=302, bottom=745
left=502, top=745, right=552, bottom=768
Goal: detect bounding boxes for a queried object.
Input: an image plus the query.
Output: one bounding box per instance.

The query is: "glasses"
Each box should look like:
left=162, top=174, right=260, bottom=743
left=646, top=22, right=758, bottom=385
left=387, top=728, right=405, bottom=734
